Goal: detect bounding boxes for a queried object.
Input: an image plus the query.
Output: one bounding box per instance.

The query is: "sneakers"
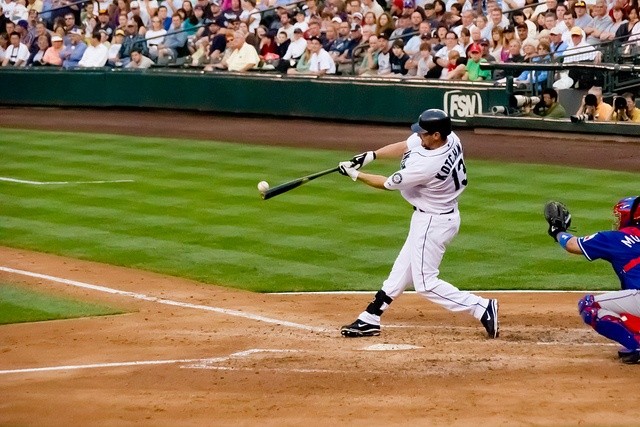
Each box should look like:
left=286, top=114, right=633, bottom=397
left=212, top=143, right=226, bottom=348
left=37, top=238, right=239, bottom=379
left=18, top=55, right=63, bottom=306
left=618, top=348, right=633, bottom=357
left=341, top=319, right=380, bottom=337
left=623, top=352, right=640, bottom=364
left=481, top=299, right=499, bottom=339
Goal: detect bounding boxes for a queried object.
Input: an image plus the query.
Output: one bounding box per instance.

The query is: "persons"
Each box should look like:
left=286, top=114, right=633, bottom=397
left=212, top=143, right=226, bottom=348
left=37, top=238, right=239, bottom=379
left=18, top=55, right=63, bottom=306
left=520, top=89, right=570, bottom=118
left=339, top=108, right=499, bottom=338
left=131, top=1, right=351, bottom=76
left=544, top=193, right=638, bottom=364
left=576, top=82, right=612, bottom=122
left=1, top=1, right=132, bottom=68
left=352, top=0, right=639, bottom=90
left=611, top=94, right=639, bottom=124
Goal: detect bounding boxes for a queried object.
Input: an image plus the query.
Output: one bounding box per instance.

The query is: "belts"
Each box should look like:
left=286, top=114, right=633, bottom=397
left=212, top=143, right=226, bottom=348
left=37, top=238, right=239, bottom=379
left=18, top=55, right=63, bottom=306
left=413, top=206, right=454, bottom=214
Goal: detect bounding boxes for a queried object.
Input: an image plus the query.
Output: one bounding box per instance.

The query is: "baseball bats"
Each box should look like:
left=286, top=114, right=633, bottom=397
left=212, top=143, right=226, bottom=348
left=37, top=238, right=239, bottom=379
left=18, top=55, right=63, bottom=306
left=259, top=159, right=358, bottom=200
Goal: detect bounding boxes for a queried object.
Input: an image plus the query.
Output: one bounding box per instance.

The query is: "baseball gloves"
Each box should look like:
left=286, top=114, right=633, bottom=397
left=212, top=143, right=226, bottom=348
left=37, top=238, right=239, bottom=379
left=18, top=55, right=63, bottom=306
left=545, top=201, right=572, bottom=235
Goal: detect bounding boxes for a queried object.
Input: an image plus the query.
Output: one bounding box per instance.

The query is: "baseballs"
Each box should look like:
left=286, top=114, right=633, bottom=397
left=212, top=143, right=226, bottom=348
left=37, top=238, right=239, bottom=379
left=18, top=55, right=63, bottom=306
left=258, top=181, right=269, bottom=192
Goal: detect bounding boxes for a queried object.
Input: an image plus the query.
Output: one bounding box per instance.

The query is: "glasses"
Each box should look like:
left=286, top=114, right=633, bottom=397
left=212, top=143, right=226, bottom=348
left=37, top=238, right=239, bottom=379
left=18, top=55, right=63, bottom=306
left=210, top=1, right=220, bottom=6
left=29, top=11, right=37, bottom=14
left=574, top=5, right=585, bottom=9
left=446, top=38, right=456, bottom=41
left=538, top=47, right=547, bottom=51
left=350, top=4, right=359, bottom=8
left=303, top=7, right=310, bottom=11
left=65, top=17, right=74, bottom=20
left=226, top=39, right=234, bottom=43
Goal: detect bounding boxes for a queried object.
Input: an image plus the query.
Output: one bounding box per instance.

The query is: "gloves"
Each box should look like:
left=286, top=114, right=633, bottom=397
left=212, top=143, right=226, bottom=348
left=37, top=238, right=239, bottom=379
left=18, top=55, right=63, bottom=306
left=350, top=151, right=376, bottom=170
left=339, top=162, right=359, bottom=181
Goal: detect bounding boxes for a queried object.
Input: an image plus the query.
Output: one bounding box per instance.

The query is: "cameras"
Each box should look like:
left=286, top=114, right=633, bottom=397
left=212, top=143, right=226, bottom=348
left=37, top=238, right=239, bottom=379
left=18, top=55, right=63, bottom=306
left=570, top=114, right=594, bottom=123
left=509, top=93, right=545, bottom=110
left=584, top=94, right=598, bottom=106
left=614, top=96, right=628, bottom=111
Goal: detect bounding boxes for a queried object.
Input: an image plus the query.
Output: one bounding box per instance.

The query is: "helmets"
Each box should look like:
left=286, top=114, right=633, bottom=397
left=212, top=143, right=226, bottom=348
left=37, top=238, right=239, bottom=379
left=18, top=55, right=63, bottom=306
left=614, top=196, right=640, bottom=230
left=411, top=109, right=451, bottom=134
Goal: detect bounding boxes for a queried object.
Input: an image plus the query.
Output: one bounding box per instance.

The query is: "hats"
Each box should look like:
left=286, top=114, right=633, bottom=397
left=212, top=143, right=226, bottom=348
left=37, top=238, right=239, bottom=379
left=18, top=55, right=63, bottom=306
left=294, top=28, right=302, bottom=33
left=378, top=32, right=387, bottom=39
left=69, top=29, right=81, bottom=36
left=422, top=33, right=431, bottom=39
left=404, top=0, right=414, bottom=7
left=424, top=4, right=435, bottom=9
left=470, top=45, right=481, bottom=53
left=276, top=5, right=286, bottom=11
left=207, top=19, right=218, bottom=25
left=127, top=21, right=137, bottom=26
left=399, top=13, right=410, bottom=18
left=332, top=16, right=342, bottom=23
left=517, top=22, right=528, bottom=28
left=115, top=30, right=123, bottom=35
left=98, top=9, right=107, bottom=15
left=18, top=21, right=28, bottom=27
left=260, top=32, right=274, bottom=39
left=349, top=24, right=361, bottom=31
left=353, top=13, right=363, bottom=19
left=311, top=36, right=322, bottom=43
left=294, top=10, right=305, bottom=15
left=571, top=27, right=582, bottom=37
left=549, top=27, right=562, bottom=35
left=480, top=38, right=489, bottom=45
left=51, top=36, right=63, bottom=41
left=131, top=1, right=138, bottom=8
left=503, top=26, right=515, bottom=33
